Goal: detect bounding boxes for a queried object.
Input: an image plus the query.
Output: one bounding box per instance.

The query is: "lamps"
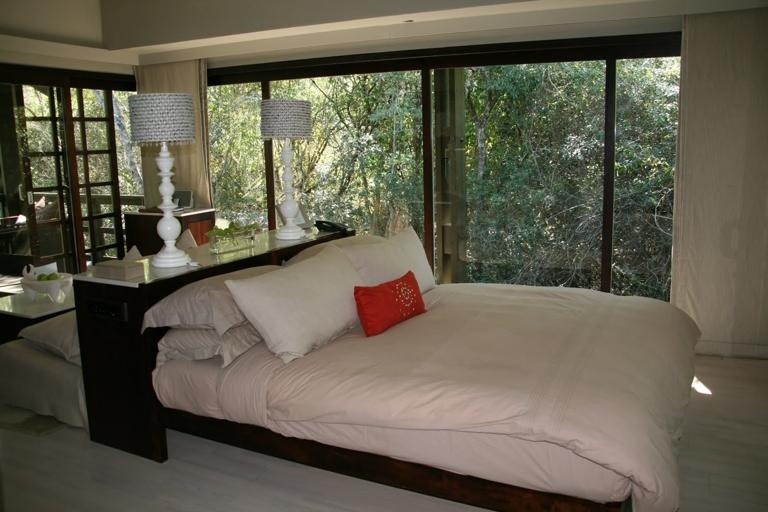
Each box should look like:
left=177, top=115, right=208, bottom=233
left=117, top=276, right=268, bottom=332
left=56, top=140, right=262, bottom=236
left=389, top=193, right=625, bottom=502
left=126, top=92, right=199, bottom=270
left=259, top=98, right=315, bottom=241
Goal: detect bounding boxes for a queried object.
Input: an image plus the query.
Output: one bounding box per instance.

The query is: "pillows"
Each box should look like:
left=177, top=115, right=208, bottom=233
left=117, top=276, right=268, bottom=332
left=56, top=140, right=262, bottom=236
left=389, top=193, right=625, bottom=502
left=21, top=308, right=82, bottom=365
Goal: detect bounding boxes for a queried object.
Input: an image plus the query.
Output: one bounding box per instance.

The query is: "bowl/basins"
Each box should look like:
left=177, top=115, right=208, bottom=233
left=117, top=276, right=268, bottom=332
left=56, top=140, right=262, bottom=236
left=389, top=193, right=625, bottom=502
left=20, top=272, right=73, bottom=302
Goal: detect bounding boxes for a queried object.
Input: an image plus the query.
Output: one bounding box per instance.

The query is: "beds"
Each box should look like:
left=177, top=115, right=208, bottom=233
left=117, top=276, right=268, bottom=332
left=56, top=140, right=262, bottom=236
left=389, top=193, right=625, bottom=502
left=73, top=225, right=704, bottom=511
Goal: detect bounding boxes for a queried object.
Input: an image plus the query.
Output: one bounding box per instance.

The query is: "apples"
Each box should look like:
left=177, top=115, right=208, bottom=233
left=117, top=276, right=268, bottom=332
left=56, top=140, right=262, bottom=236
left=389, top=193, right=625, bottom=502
left=48, top=273, right=57, bottom=280
left=37, top=273, right=48, bottom=280
